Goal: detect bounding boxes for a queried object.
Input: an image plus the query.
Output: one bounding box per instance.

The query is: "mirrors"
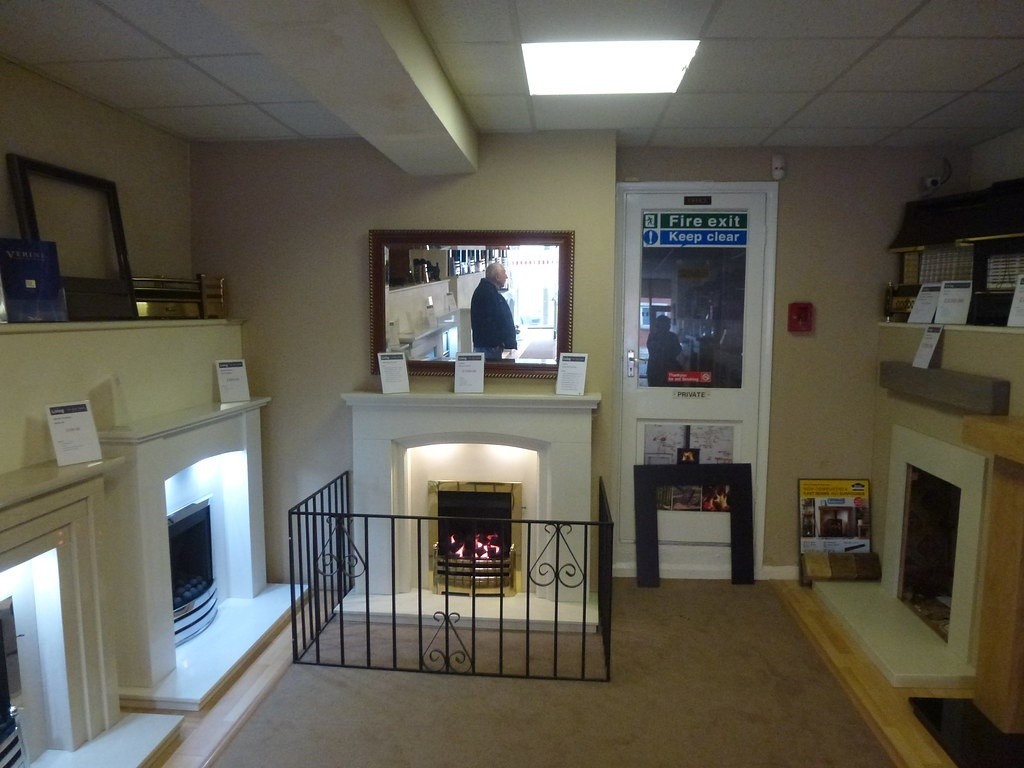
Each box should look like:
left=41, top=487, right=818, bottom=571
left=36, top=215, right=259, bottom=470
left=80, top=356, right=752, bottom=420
left=384, top=243, right=558, bottom=365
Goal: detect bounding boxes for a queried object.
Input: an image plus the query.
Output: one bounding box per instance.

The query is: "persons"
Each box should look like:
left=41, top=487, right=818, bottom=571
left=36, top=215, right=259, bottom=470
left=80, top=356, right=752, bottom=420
left=645, top=314, right=683, bottom=385
left=470, top=263, right=517, bottom=360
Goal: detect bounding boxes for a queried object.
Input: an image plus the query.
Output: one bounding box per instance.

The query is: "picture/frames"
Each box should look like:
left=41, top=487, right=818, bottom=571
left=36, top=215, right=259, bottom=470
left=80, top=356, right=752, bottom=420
left=6, top=153, right=137, bottom=320
